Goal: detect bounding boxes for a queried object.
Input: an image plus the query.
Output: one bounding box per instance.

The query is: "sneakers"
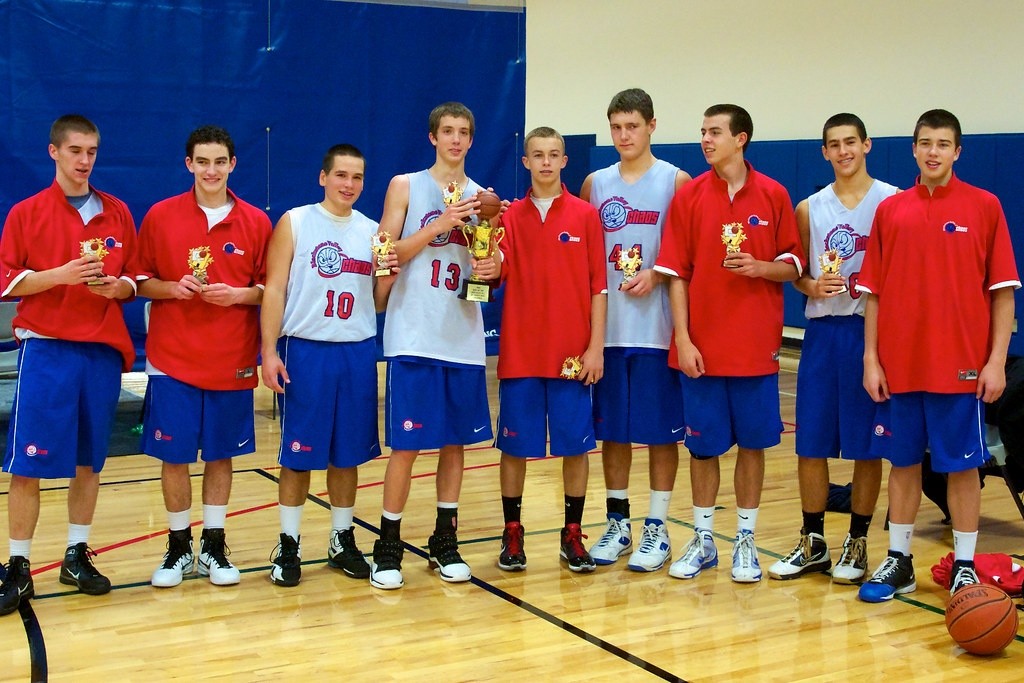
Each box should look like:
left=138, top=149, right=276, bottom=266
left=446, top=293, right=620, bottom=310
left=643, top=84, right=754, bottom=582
left=327, top=526, right=371, bottom=577
left=769, top=526, right=832, bottom=580
left=498, top=521, right=527, bottom=570
left=949, top=560, right=980, bottom=596
left=60, top=542, right=111, bottom=594
left=627, top=518, right=671, bottom=570
left=560, top=524, right=596, bottom=572
left=731, top=529, right=762, bottom=581
left=152, top=534, right=195, bottom=586
left=860, top=550, right=917, bottom=602
left=589, top=513, right=633, bottom=564
left=668, top=528, right=718, bottom=578
left=429, top=533, right=471, bottom=582
left=0, top=556, right=33, bottom=614
left=369, top=539, right=405, bottom=588
left=270, top=533, right=301, bottom=586
left=197, top=531, right=240, bottom=585
left=832, top=533, right=868, bottom=584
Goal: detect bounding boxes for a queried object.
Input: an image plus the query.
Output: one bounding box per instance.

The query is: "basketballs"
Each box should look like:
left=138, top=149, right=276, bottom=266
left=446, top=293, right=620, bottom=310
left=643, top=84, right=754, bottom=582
left=944, top=582, right=1020, bottom=656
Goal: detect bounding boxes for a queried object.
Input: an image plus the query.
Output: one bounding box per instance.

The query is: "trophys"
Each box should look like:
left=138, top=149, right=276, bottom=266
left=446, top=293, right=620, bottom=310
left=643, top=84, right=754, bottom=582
left=720, top=222, right=747, bottom=268
left=442, top=181, right=472, bottom=228
left=818, top=249, right=848, bottom=296
left=617, top=247, right=643, bottom=290
left=456, top=219, right=505, bottom=302
left=561, top=355, right=595, bottom=382
left=80, top=238, right=107, bottom=285
left=370, top=231, right=396, bottom=276
left=186, top=245, right=214, bottom=290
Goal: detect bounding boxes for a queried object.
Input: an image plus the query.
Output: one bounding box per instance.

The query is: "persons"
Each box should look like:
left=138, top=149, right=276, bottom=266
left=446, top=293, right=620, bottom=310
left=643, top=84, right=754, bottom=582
left=498, top=88, right=694, bottom=574
left=262, top=142, right=401, bottom=588
left=135, top=126, right=273, bottom=588
left=653, top=104, right=808, bottom=583
left=470, top=127, right=607, bottom=575
left=369, top=101, right=500, bottom=591
left=0, top=111, right=139, bottom=617
left=765, top=114, right=905, bottom=586
left=858, top=110, right=1022, bottom=604
